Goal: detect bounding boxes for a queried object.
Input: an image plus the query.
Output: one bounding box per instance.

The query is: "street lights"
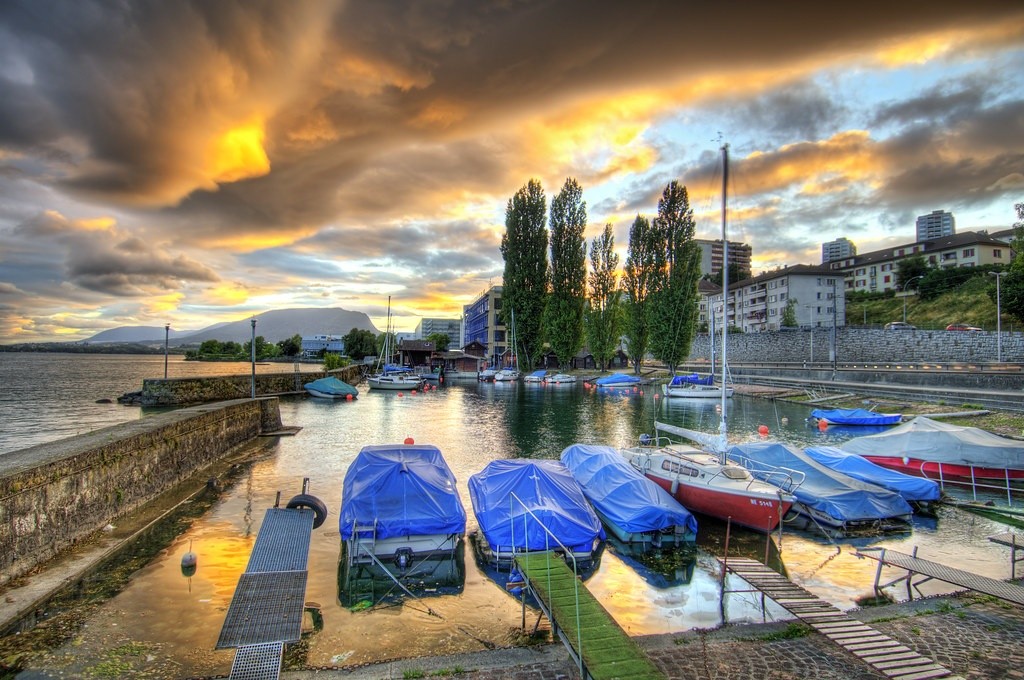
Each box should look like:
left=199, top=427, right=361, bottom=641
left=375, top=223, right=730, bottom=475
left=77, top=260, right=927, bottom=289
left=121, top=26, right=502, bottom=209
left=806, top=305, right=818, bottom=362
left=251, top=319, right=258, bottom=399
left=903, top=275, right=924, bottom=322
left=165, top=325, right=170, bottom=379
left=987, top=270, right=1008, bottom=363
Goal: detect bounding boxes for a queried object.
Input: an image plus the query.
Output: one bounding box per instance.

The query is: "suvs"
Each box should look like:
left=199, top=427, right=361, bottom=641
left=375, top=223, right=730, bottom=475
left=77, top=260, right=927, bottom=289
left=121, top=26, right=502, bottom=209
left=883, top=322, right=918, bottom=330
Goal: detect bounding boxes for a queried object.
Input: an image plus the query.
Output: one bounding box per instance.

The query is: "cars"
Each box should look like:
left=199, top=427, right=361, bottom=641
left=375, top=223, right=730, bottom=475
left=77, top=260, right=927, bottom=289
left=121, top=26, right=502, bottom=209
left=945, top=324, right=983, bottom=331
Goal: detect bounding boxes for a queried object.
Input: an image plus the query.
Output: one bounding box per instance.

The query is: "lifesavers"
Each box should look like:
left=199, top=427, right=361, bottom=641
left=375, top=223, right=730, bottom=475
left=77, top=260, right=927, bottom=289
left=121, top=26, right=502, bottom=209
left=286, top=494, right=327, bottom=528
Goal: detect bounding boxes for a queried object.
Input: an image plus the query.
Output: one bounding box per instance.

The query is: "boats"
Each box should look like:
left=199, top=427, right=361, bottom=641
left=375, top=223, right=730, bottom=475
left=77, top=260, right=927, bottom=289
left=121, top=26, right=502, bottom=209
left=338, top=438, right=466, bottom=594
left=804, top=445, right=944, bottom=520
left=481, top=367, right=716, bottom=387
left=303, top=376, right=359, bottom=399
left=811, top=408, right=903, bottom=426
left=467, top=458, right=607, bottom=579
left=725, top=440, right=913, bottom=542
left=832, top=416, right=1024, bottom=497
left=558, top=444, right=698, bottom=555
left=420, top=373, right=439, bottom=379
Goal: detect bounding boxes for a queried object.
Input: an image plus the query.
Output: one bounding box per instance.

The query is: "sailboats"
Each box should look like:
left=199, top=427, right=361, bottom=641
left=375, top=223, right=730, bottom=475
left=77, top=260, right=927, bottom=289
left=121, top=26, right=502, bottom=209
left=623, top=133, right=806, bottom=534
left=662, top=299, right=734, bottom=397
left=367, top=296, right=427, bottom=390
